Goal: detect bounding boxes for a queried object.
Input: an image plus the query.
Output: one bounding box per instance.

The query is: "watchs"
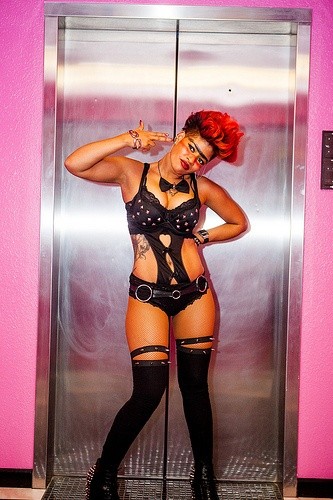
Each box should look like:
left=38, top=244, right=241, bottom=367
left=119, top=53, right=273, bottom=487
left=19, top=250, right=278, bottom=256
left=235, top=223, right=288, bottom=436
left=198, top=229, right=210, bottom=244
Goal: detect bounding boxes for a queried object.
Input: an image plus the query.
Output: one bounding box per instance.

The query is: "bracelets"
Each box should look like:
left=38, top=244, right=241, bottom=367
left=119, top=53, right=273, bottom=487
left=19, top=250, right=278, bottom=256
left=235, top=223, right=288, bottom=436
left=128, top=128, right=142, bottom=150
left=192, top=233, right=202, bottom=247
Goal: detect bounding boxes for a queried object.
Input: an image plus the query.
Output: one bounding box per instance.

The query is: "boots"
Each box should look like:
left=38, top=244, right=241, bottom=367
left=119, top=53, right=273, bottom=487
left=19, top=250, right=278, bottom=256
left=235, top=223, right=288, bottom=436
left=175, top=335, right=219, bottom=500
left=83, top=345, right=171, bottom=500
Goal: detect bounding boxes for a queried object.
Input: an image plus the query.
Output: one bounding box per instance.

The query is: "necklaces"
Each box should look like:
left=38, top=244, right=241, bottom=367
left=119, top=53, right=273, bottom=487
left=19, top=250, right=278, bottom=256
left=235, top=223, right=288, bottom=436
left=157, top=159, right=191, bottom=195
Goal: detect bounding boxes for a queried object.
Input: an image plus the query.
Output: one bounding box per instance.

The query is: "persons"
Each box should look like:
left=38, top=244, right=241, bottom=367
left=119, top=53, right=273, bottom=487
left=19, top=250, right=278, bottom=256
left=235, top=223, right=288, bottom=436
left=63, top=109, right=249, bottom=500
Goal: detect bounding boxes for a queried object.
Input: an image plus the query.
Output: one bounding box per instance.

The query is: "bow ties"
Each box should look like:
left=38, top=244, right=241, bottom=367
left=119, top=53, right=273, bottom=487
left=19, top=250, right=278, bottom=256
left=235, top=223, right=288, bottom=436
left=159, top=177, right=189, bottom=195
